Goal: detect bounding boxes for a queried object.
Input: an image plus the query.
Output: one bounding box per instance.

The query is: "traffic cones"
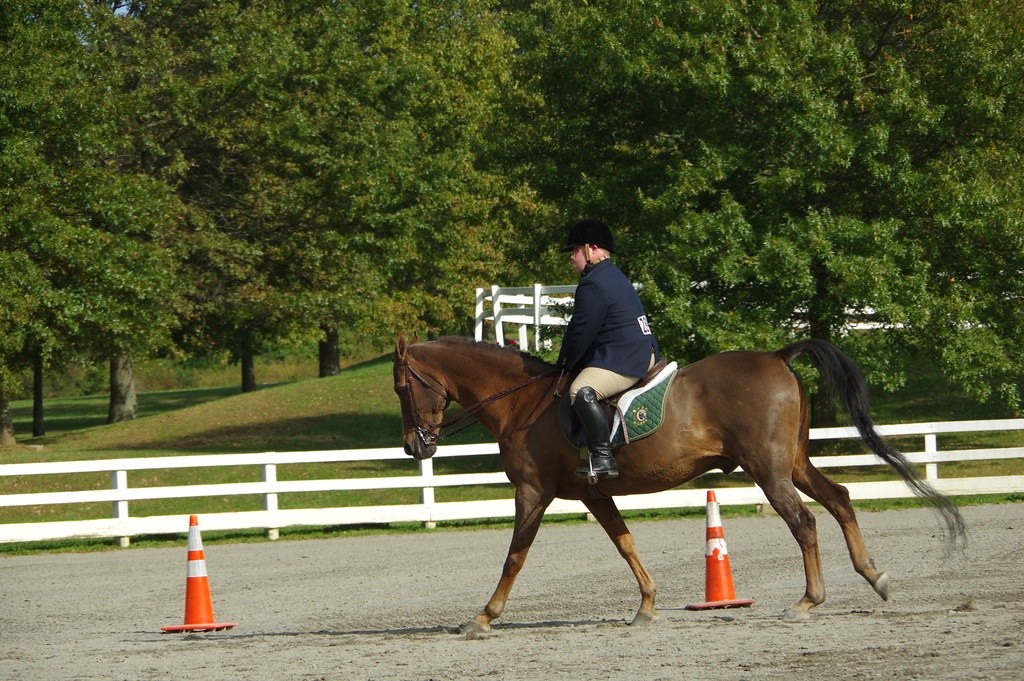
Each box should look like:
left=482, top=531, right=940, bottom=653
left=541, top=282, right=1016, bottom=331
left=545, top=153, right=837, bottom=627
left=160, top=513, right=237, bottom=632
left=685, top=490, right=756, bottom=610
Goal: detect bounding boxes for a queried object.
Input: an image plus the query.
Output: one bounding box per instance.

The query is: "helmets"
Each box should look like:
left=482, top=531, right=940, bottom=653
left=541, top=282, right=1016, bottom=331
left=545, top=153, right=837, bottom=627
left=560, top=220, right=615, bottom=253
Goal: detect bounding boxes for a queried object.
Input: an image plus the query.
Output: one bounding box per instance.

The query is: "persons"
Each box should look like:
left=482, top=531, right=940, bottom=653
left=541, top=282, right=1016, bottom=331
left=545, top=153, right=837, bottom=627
left=556, top=219, right=661, bottom=480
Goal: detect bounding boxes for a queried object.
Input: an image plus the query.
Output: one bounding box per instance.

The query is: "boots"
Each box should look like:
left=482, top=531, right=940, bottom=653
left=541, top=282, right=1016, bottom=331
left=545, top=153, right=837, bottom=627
left=570, top=386, right=620, bottom=480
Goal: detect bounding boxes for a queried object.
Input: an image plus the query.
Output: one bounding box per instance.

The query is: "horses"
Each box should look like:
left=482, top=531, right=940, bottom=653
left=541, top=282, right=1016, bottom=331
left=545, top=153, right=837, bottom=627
left=391, top=331, right=969, bottom=635
